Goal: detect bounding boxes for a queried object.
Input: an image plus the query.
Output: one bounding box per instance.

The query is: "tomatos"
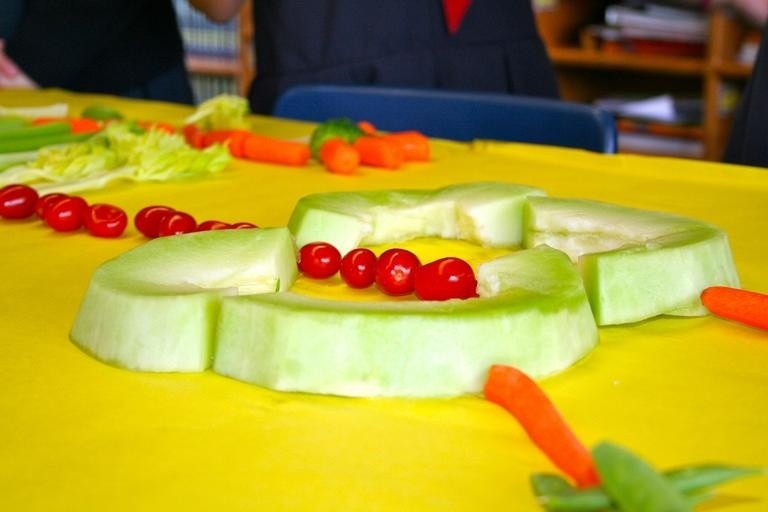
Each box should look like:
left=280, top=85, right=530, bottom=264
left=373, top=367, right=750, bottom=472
left=0, top=185, right=479, bottom=300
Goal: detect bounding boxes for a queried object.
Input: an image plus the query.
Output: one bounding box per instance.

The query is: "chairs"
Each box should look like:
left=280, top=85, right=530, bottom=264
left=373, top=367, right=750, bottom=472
left=271, top=83, right=618, bottom=154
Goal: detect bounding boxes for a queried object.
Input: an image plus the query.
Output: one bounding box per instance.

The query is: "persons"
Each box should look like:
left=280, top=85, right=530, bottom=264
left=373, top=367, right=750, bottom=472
left=0, top=1, right=241, bottom=109
left=246, top=0, right=561, bottom=115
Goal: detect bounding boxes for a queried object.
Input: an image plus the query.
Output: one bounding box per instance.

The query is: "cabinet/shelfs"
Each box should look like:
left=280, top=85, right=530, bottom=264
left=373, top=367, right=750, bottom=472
left=173, top=0, right=259, bottom=106
left=527, top=1, right=768, bottom=156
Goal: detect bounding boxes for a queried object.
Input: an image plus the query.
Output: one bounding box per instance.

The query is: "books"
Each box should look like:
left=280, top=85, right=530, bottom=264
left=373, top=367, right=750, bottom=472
left=173, top=1, right=238, bottom=105
left=583, top=1, right=708, bottom=159
